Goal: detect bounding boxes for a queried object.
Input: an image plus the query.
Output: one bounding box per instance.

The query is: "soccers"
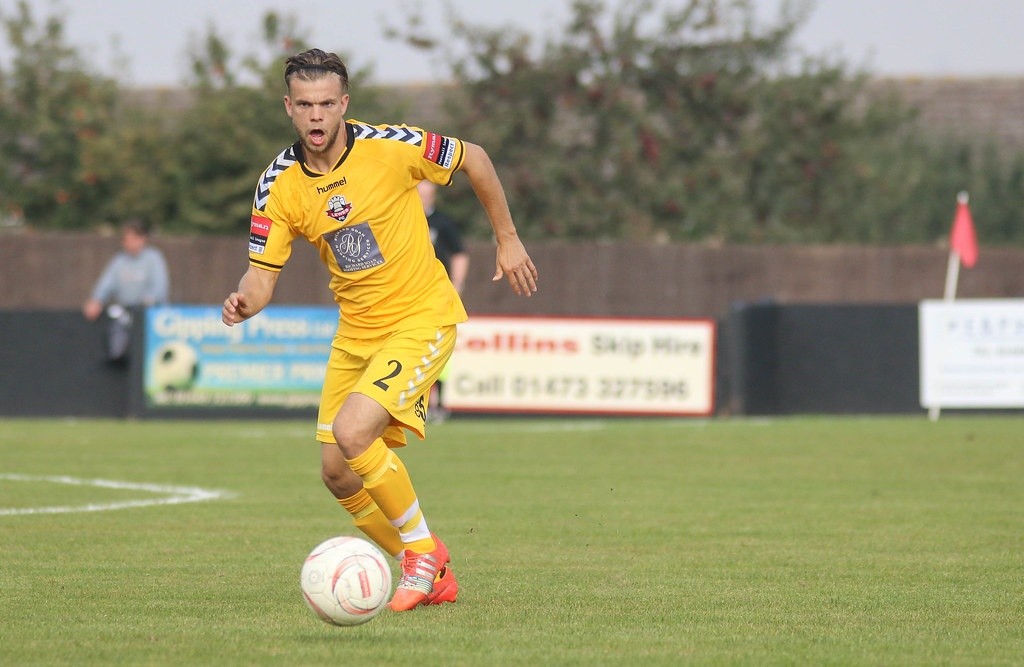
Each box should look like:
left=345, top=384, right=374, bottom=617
left=152, top=342, right=199, bottom=392
left=301, top=536, right=392, bottom=627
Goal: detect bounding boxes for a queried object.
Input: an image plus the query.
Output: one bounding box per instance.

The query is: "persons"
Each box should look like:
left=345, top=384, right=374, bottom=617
left=222, top=50, right=538, bottom=612
left=84, top=217, right=169, bottom=363
left=416, top=179, right=471, bottom=420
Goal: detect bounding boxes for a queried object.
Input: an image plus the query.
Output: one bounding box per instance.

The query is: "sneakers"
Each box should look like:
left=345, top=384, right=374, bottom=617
left=387, top=566, right=458, bottom=610
left=391, top=532, right=451, bottom=611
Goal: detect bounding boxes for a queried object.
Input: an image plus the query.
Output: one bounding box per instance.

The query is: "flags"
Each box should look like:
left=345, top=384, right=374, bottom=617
left=952, top=204, right=978, bottom=268
